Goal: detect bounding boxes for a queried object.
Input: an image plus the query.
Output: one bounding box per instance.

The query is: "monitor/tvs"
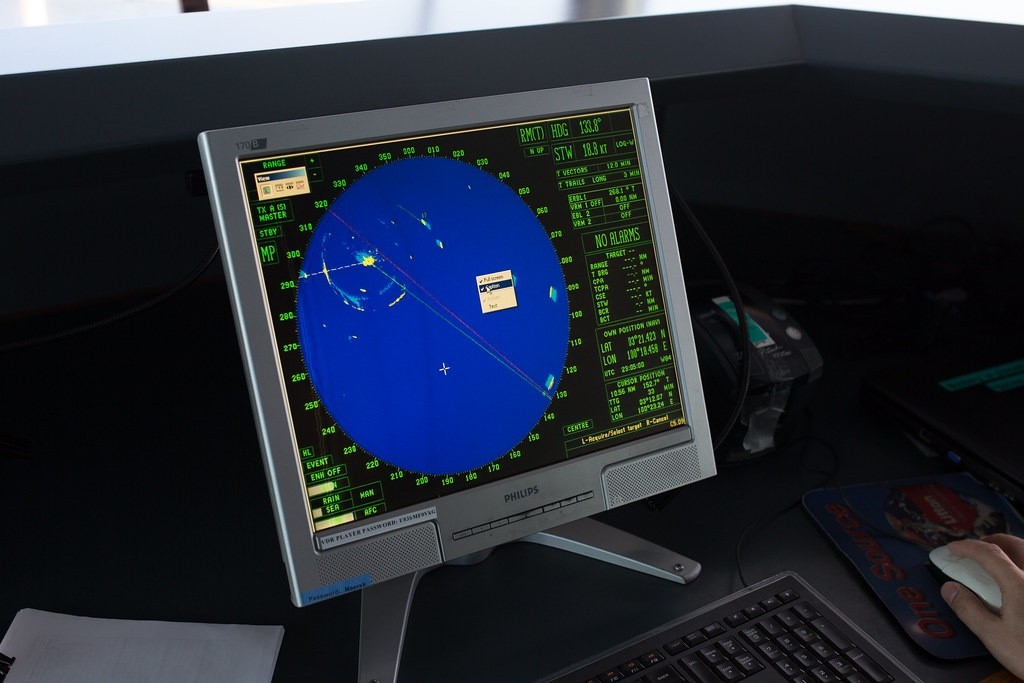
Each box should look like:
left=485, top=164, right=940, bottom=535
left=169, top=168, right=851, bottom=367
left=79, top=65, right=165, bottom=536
left=197, top=75, right=720, bottom=611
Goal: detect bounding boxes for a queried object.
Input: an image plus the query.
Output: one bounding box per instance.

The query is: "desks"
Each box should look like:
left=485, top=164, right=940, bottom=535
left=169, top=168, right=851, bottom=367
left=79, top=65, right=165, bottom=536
left=1, top=428, right=1022, bottom=682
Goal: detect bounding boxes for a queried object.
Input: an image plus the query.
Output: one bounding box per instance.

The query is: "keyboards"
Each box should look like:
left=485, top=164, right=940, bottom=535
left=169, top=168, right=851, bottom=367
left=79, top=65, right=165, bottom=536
left=528, top=570, right=923, bottom=683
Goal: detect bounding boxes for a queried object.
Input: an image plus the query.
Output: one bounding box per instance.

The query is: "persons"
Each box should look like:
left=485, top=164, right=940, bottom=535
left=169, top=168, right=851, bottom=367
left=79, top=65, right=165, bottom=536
left=941, top=531, right=1024, bottom=682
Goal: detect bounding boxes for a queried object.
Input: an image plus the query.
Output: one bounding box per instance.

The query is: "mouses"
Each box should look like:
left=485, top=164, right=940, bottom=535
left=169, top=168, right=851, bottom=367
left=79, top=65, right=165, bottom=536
left=929, top=539, right=1007, bottom=616
